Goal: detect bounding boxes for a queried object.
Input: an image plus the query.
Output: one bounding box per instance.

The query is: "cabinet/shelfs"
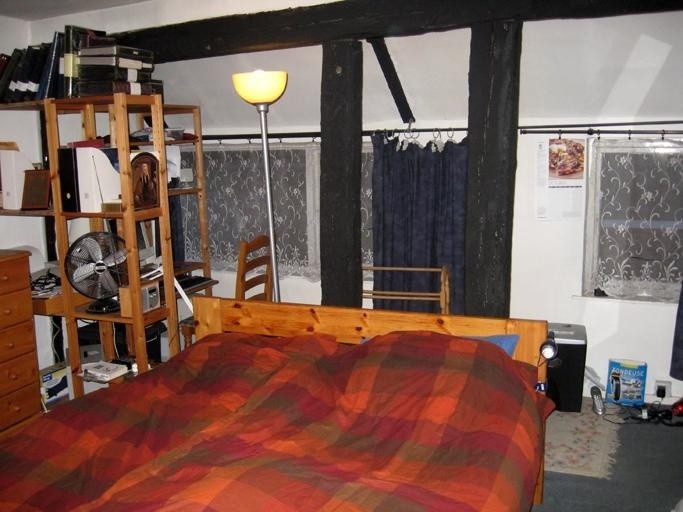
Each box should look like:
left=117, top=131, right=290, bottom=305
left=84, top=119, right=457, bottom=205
left=0, top=248, right=44, bottom=442
left=1, top=94, right=213, bottom=397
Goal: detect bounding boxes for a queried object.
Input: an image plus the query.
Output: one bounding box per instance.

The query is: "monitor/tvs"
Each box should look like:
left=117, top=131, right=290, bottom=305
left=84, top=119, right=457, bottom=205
left=104, top=217, right=153, bottom=263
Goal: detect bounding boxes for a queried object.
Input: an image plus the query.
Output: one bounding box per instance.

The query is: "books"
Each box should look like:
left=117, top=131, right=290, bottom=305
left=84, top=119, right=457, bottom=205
left=1, top=23, right=157, bottom=104
left=85, top=360, right=128, bottom=380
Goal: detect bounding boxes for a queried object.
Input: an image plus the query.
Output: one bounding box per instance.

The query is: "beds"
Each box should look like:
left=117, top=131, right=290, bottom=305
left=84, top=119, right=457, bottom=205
left=0, top=294, right=549, bottom=512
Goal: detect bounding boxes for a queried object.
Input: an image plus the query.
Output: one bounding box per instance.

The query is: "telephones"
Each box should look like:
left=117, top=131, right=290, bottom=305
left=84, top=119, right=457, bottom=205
left=590, top=386, right=605, bottom=415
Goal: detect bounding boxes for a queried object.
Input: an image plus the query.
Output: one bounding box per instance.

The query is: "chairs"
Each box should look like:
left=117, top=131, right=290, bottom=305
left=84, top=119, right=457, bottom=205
left=179, top=236, right=275, bottom=352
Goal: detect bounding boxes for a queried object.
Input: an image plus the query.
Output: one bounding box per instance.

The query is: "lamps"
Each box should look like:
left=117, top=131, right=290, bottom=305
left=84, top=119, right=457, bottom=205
left=231, top=67, right=288, bottom=305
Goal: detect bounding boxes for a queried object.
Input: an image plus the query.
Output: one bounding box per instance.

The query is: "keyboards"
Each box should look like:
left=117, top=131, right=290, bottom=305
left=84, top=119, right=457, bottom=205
left=175, top=275, right=213, bottom=294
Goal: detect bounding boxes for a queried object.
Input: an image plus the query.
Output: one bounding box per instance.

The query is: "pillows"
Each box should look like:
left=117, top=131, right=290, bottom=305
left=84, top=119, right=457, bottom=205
left=455, top=334, right=520, bottom=360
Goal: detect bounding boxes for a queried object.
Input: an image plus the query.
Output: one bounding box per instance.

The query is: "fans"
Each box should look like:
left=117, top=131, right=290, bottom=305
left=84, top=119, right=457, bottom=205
left=63, top=231, right=129, bottom=315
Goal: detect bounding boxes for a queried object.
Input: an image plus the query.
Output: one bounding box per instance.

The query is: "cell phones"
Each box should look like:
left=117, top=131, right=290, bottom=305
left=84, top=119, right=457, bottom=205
left=590, top=386, right=605, bottom=412
